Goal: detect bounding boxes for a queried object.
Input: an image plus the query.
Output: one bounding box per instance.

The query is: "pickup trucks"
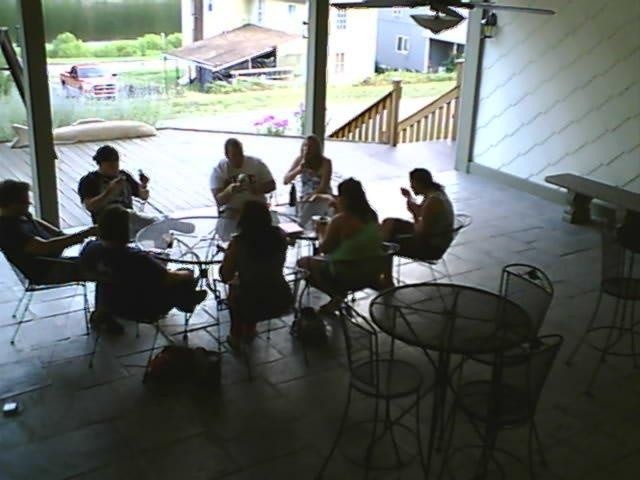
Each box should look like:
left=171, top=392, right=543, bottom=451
left=60, top=63, right=118, bottom=99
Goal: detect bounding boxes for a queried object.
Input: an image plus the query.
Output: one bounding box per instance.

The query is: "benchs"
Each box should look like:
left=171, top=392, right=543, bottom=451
left=545, top=173, right=640, bottom=225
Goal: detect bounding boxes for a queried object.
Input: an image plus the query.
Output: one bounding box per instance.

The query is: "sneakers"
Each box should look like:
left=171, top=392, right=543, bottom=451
left=175, top=289, right=206, bottom=314
left=167, top=221, right=195, bottom=234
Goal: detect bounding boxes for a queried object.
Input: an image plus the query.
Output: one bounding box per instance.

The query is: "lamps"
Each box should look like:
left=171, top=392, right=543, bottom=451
left=479, top=12, right=496, bottom=38
left=410, top=14, right=462, bottom=32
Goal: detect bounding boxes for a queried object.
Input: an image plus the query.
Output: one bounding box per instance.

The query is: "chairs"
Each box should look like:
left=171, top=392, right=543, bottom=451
left=566, top=224, right=640, bottom=372
left=297, top=242, right=400, bottom=313
left=87, top=269, right=196, bottom=381
left=129, top=196, right=168, bottom=248
left=393, top=214, right=472, bottom=309
left=320, top=299, right=425, bottom=480
left=439, top=335, right=562, bottom=480
left=213, top=266, right=311, bottom=374
left=0, top=246, right=95, bottom=343
left=460, top=264, right=554, bottom=381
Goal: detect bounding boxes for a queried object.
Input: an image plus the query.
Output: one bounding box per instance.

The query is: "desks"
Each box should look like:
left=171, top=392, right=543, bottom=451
left=371, top=282, right=529, bottom=480
left=136, top=216, right=241, bottom=338
left=272, top=197, right=336, bottom=256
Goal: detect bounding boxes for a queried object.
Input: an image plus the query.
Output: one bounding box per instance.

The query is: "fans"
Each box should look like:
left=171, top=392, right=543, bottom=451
left=329, top=0, right=554, bottom=19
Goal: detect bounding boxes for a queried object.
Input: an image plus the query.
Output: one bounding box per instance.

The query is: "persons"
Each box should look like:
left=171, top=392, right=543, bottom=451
left=382, top=168, right=454, bottom=288
left=80, top=204, right=207, bottom=322
left=0, top=181, right=125, bottom=333
left=220, top=199, right=288, bottom=349
left=297, top=178, right=384, bottom=313
left=78, top=146, right=149, bottom=228
left=284, top=134, right=334, bottom=208
left=211, top=138, right=276, bottom=210
